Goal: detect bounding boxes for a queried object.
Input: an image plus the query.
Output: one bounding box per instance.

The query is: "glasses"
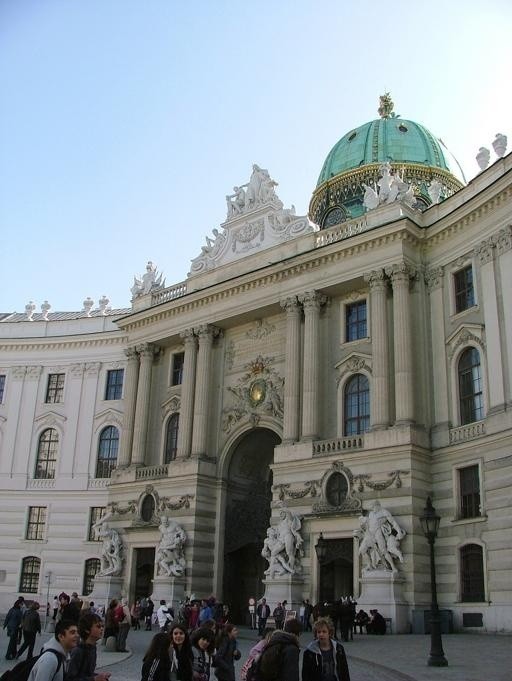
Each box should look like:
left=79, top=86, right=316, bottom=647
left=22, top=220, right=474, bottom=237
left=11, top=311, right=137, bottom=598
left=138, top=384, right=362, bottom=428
left=96, top=624, right=105, bottom=628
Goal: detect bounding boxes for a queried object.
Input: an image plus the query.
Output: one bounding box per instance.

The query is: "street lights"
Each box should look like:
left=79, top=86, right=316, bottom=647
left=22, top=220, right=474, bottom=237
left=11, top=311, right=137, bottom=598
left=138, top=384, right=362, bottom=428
left=315, top=531, right=329, bottom=600
left=418, top=493, right=448, bottom=667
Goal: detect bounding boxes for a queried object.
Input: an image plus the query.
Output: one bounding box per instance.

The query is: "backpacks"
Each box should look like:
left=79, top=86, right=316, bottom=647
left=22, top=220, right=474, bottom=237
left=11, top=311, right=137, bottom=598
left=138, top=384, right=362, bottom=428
left=0, top=647, right=61, bottom=681
left=115, top=605, right=127, bottom=622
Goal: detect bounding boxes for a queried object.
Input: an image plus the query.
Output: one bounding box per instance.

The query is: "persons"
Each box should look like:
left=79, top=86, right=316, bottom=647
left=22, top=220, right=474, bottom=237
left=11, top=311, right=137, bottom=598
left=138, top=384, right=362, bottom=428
left=46, top=591, right=96, bottom=621
left=25, top=620, right=79, bottom=680
left=190, top=229, right=225, bottom=262
left=2, top=597, right=42, bottom=660
left=229, top=186, right=245, bottom=213
left=102, top=598, right=119, bottom=647
left=274, top=600, right=288, bottom=629
left=94, top=523, right=123, bottom=578
left=300, top=618, right=350, bottom=681
left=255, top=597, right=270, bottom=635
left=116, top=597, right=132, bottom=652
left=260, top=508, right=301, bottom=582
left=252, top=620, right=304, bottom=680
left=240, top=628, right=275, bottom=679
left=152, top=515, right=185, bottom=580
left=360, top=500, right=402, bottom=575
left=298, top=594, right=386, bottom=642
left=132, top=595, right=242, bottom=681
left=67, top=613, right=112, bottom=680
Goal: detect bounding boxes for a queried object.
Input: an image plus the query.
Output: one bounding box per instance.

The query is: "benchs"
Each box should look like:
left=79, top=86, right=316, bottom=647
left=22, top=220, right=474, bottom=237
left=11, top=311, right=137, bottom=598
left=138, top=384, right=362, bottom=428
left=368, top=618, right=392, bottom=634
